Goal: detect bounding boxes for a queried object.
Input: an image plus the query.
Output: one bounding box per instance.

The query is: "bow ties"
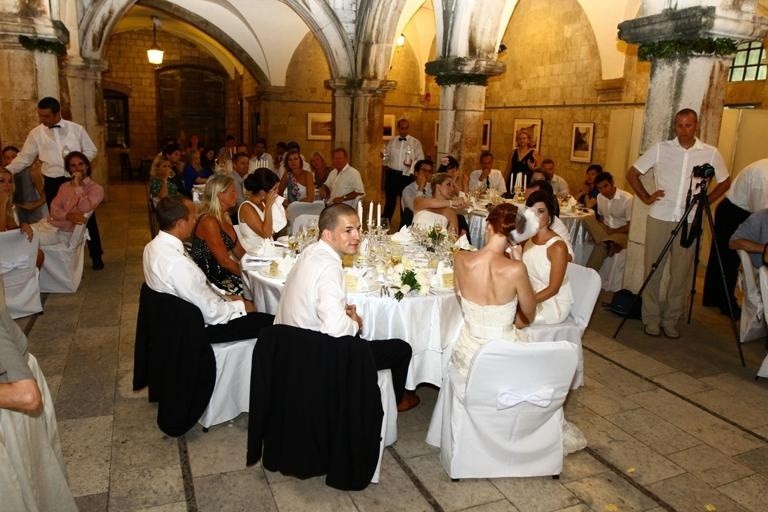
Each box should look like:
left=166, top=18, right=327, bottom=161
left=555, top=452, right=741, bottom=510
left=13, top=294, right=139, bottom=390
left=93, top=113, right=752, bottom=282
left=399, top=138, right=406, bottom=141
left=49, top=125, right=60, bottom=128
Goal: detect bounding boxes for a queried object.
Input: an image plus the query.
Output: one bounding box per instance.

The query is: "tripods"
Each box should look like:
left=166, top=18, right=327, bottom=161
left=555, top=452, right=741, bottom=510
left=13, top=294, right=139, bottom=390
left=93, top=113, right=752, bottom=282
left=612, top=194, right=746, bottom=369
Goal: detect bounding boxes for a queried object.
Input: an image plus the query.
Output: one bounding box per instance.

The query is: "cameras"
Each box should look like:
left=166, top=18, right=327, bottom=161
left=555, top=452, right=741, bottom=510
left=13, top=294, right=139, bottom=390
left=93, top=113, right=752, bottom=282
left=694, top=164, right=716, bottom=178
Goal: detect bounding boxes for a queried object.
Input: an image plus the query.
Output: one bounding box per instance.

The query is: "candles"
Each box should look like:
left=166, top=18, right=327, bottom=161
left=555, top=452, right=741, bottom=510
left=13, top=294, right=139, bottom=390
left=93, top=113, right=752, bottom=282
left=510, top=173, right=527, bottom=193
left=368, top=200, right=382, bottom=232
left=254, top=158, right=269, bottom=170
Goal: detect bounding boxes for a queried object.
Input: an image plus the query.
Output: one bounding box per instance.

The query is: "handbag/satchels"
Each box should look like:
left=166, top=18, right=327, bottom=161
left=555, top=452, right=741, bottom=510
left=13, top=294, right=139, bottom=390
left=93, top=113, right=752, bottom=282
left=611, top=289, right=642, bottom=320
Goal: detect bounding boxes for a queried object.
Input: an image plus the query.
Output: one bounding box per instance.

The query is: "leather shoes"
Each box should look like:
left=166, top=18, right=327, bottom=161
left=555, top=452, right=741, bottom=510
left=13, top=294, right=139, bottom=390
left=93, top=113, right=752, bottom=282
left=645, top=324, right=661, bottom=336
left=664, top=327, right=680, bottom=338
left=396, top=391, right=420, bottom=411
left=607, top=243, right=623, bottom=257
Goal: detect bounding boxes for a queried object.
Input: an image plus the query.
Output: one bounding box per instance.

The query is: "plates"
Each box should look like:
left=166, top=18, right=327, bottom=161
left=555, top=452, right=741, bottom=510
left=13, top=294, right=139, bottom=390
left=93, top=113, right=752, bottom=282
left=258, top=265, right=284, bottom=280
left=359, top=282, right=381, bottom=295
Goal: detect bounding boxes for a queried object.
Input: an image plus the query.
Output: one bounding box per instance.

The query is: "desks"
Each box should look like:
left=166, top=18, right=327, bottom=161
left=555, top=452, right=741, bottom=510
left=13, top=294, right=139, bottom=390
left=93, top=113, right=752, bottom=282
left=452, top=192, right=595, bottom=236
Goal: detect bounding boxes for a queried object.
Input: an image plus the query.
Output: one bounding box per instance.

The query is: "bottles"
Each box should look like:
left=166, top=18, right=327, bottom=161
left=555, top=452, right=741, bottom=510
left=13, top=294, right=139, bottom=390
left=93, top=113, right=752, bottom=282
left=404, top=144, right=413, bottom=165
left=382, top=144, right=392, bottom=167
left=61, top=144, right=70, bottom=159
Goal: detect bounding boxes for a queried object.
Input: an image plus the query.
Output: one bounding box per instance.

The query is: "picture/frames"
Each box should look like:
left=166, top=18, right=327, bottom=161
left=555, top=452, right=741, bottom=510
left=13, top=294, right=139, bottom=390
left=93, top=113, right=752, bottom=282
left=480, top=119, right=491, bottom=152
left=569, top=122, right=594, bottom=164
left=381, top=114, right=396, bottom=140
left=306, top=112, right=332, bottom=141
left=511, top=118, right=542, bottom=156
left=434, top=120, right=439, bottom=147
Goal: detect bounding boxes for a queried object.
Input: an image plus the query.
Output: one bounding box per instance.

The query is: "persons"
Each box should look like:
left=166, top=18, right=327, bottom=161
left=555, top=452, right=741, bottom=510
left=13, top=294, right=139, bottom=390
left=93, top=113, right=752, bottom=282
left=0, top=273, right=80, bottom=512
left=504, top=130, right=540, bottom=198
left=727, top=208, right=768, bottom=383
left=152, top=135, right=366, bottom=292
left=625, top=109, right=732, bottom=339
left=275, top=203, right=420, bottom=411
left=453, top=203, right=587, bottom=454
left=701, top=158, right=768, bottom=321
left=519, top=185, right=572, bottom=324
left=381, top=120, right=424, bottom=230
left=401, top=152, right=633, bottom=272
left=143, top=195, right=275, bottom=344
left=0, top=96, right=106, bottom=270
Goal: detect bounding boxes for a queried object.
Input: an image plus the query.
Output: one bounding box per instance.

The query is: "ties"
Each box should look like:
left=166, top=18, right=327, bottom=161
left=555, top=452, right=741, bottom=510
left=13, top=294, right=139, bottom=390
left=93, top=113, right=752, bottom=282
left=486, top=176, right=489, bottom=188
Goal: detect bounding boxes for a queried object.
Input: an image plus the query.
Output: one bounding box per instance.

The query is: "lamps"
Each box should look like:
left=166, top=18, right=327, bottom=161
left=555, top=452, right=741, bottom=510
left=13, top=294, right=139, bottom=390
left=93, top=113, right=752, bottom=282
left=147, top=14, right=165, bottom=72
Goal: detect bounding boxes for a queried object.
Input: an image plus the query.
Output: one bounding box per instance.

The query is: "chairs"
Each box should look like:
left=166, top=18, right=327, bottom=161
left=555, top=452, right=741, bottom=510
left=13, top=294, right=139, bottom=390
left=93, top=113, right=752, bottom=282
left=0, top=351, right=82, bottom=512
left=755, top=265, right=768, bottom=381
left=735, top=248, right=768, bottom=345
left=245, top=324, right=398, bottom=489
left=144, top=182, right=160, bottom=240
left=0, top=226, right=43, bottom=322
left=133, top=283, right=259, bottom=433
left=438, top=340, right=581, bottom=481
left=192, top=176, right=366, bottom=234
left=517, top=261, right=603, bottom=343
left=38, top=209, right=96, bottom=293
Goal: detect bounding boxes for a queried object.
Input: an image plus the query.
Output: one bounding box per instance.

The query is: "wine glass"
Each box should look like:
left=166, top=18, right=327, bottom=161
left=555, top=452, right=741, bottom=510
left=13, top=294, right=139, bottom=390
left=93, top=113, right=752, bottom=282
left=365, top=216, right=377, bottom=232
left=287, top=218, right=319, bottom=265
left=556, top=191, right=591, bottom=215
left=381, top=217, right=391, bottom=235
left=364, top=233, right=404, bottom=269
left=412, top=221, right=461, bottom=268
left=463, top=184, right=506, bottom=213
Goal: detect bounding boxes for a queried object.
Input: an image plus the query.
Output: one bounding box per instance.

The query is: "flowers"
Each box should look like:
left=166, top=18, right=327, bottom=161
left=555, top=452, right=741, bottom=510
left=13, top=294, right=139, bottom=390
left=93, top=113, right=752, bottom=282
left=387, top=260, right=432, bottom=301
